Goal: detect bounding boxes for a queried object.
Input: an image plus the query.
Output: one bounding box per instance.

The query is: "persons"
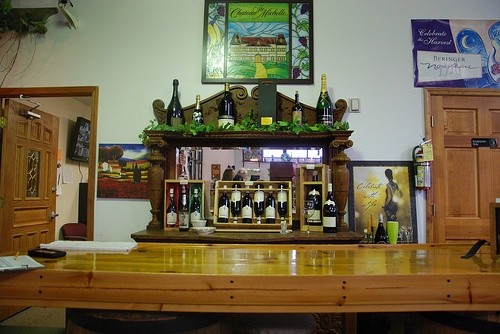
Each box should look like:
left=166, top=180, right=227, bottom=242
left=233, top=168, right=248, bottom=181
left=382, top=169, right=403, bottom=221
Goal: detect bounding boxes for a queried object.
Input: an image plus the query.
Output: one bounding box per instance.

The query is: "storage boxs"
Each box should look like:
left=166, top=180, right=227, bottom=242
left=299, top=163, right=329, bottom=232
left=163, top=180, right=205, bottom=229
left=213, top=180, right=293, bottom=225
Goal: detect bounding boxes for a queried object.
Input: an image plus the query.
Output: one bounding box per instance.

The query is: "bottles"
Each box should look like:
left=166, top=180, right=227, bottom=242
left=190, top=189, right=201, bottom=228
left=192, top=94, right=204, bottom=125
left=242, top=185, right=253, bottom=224
left=315, top=74, right=333, bottom=127
left=277, top=185, right=287, bottom=221
left=178, top=185, right=189, bottom=232
left=374, top=214, right=387, bottom=244
left=322, top=182, right=337, bottom=233
left=265, top=184, right=276, bottom=224
left=217, top=82, right=234, bottom=130
left=218, top=185, right=230, bottom=223
left=291, top=92, right=303, bottom=126
left=166, top=79, right=184, bottom=126
left=254, top=184, right=266, bottom=224
left=360, top=229, right=371, bottom=244
left=230, top=184, right=242, bottom=223
left=305, top=171, right=322, bottom=226
left=166, top=188, right=177, bottom=228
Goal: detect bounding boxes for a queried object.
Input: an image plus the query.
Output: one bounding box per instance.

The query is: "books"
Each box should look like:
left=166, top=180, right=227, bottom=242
left=0, top=256, right=44, bottom=272
left=39, top=241, right=138, bottom=252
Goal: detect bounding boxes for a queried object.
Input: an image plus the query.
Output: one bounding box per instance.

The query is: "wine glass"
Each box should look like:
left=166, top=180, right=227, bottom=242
left=301, top=201, right=315, bottom=235
left=398, top=226, right=413, bottom=244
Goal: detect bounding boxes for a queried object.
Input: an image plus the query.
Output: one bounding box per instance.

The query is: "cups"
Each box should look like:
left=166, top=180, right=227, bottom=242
left=190, top=220, right=207, bottom=227
left=387, top=221, right=398, bottom=244
left=194, top=227, right=215, bottom=236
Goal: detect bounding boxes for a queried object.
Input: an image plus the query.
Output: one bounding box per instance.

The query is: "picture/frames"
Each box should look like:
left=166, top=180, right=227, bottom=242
left=347, top=161, right=421, bottom=244
left=201, top=0, right=314, bottom=84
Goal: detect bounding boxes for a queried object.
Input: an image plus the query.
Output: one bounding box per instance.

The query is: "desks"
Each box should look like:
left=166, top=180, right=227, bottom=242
left=0, top=240, right=499, bottom=312
